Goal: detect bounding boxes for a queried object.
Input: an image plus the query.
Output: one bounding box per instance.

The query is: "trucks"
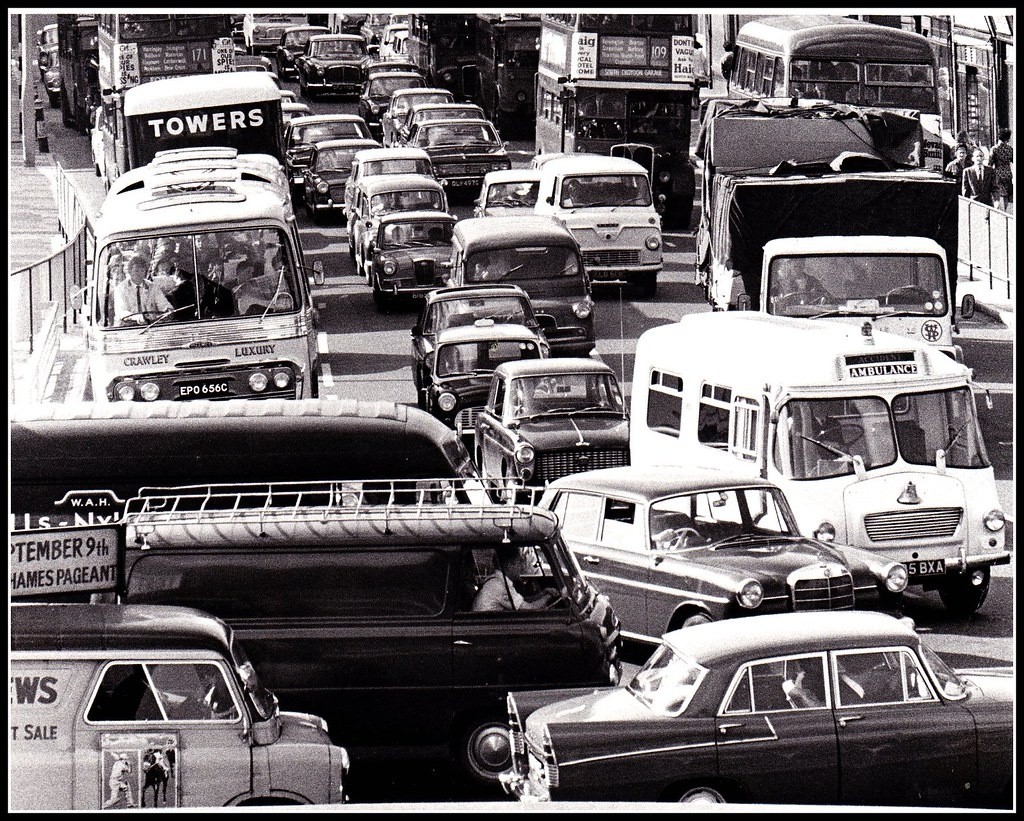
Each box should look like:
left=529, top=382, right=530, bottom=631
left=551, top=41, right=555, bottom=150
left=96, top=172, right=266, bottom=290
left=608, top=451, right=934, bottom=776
left=691, top=96, right=977, bottom=364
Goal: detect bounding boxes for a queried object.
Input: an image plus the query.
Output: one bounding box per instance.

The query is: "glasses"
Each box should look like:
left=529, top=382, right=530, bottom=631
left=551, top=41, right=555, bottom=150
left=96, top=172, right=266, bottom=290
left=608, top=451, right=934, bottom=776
left=512, top=562, right=523, bottom=567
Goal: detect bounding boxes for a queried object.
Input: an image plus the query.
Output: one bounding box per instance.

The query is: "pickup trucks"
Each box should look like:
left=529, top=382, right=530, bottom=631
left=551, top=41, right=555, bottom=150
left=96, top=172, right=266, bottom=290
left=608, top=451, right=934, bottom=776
left=493, top=610, right=1015, bottom=812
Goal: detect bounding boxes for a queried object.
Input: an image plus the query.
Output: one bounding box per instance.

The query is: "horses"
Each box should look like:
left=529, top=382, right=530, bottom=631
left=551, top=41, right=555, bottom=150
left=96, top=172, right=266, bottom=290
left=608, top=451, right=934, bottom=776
left=141, top=752, right=169, bottom=807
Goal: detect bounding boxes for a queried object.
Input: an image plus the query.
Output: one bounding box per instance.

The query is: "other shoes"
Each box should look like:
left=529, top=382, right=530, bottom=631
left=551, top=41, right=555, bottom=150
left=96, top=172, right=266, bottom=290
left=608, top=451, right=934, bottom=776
left=130, top=804, right=138, bottom=807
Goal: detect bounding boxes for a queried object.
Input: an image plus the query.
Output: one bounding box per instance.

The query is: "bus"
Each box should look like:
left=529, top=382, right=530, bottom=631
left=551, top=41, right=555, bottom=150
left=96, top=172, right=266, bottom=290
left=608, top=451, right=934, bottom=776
left=37, top=12, right=947, bottom=310
left=69, top=147, right=327, bottom=401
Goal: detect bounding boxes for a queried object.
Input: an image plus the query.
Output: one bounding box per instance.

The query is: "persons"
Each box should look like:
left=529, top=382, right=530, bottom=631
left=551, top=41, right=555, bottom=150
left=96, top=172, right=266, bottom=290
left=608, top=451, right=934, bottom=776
left=811, top=400, right=850, bottom=461
left=107, top=229, right=285, bottom=327
left=788, top=61, right=933, bottom=104
left=987, top=128, right=1012, bottom=212
left=563, top=179, right=581, bottom=207
left=473, top=547, right=559, bottom=612
left=962, top=148, right=1000, bottom=210
left=771, top=257, right=831, bottom=304
left=103, top=752, right=137, bottom=808
left=721, top=41, right=734, bottom=94
left=945, top=143, right=974, bottom=183
left=691, top=41, right=709, bottom=109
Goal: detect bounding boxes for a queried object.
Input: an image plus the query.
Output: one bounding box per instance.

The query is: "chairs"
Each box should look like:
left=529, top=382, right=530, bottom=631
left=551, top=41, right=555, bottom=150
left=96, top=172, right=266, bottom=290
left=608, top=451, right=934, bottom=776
left=788, top=687, right=823, bottom=707
left=782, top=681, right=799, bottom=709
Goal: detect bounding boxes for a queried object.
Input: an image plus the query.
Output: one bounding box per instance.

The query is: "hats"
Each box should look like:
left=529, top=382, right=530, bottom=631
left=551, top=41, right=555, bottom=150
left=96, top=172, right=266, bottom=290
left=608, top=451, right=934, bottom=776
left=120, top=753, right=130, bottom=759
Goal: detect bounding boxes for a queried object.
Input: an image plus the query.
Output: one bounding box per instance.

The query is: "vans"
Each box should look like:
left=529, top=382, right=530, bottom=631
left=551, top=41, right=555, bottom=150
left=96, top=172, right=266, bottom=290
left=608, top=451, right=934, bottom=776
left=629, top=310, right=1010, bottom=616
left=9, top=602, right=348, bottom=811
left=444, top=214, right=596, bottom=356
left=8, top=398, right=492, bottom=537
left=86, top=476, right=624, bottom=786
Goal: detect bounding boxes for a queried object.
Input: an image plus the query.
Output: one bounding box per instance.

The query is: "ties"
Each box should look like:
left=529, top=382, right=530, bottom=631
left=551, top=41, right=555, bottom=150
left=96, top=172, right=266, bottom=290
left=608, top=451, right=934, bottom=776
left=136, top=286, right=142, bottom=313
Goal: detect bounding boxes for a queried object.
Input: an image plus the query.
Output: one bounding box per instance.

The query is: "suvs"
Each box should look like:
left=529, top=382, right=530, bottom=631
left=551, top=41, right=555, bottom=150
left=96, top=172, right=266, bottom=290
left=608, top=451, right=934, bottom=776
left=410, top=283, right=557, bottom=457
left=473, top=357, right=629, bottom=505
left=534, top=463, right=908, bottom=666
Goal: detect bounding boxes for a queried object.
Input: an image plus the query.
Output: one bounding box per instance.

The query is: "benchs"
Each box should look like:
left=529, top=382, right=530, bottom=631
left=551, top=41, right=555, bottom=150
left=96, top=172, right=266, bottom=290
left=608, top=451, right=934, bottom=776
left=615, top=512, right=691, bottom=532
